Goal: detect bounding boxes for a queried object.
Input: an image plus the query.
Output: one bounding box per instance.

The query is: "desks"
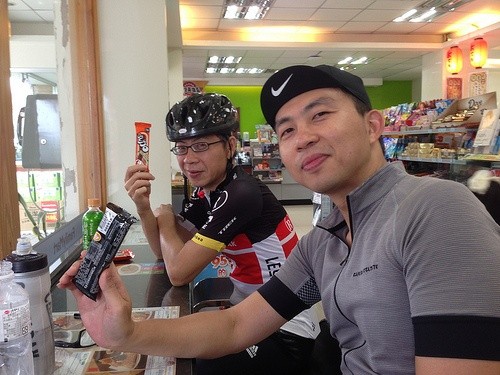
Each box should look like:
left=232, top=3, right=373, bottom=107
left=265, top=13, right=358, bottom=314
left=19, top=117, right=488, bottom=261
left=50, top=220, right=193, bottom=375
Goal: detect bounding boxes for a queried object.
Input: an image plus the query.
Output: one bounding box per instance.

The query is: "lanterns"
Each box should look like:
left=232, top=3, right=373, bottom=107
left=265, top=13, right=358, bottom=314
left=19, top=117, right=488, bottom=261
left=470, top=37, right=489, bottom=69
left=446, top=46, right=464, bottom=75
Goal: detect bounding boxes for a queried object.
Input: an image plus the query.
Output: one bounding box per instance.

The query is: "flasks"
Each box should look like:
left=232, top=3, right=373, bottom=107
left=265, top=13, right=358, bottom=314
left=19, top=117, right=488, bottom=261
left=3, top=237, right=55, bottom=375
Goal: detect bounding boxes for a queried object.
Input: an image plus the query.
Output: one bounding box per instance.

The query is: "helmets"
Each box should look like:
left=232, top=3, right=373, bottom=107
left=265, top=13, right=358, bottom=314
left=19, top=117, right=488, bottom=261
left=165, top=93, right=239, bottom=140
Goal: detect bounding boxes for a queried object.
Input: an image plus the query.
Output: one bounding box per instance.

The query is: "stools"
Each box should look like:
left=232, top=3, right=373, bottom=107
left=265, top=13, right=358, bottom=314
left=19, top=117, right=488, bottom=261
left=192, top=277, right=234, bottom=313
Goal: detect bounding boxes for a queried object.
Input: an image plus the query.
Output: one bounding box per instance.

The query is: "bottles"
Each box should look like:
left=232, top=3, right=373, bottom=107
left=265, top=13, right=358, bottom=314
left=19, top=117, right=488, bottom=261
left=0, top=260, right=34, bottom=375
left=82, top=198, right=104, bottom=249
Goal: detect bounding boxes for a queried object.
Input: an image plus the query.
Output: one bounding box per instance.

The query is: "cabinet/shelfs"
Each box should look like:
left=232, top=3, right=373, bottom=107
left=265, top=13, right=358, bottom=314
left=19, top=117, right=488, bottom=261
left=250, top=92, right=500, bottom=201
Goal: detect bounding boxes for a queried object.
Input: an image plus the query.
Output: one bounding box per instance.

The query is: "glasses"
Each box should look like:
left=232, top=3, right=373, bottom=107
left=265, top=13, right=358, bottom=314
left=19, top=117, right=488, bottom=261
left=169, top=140, right=229, bottom=156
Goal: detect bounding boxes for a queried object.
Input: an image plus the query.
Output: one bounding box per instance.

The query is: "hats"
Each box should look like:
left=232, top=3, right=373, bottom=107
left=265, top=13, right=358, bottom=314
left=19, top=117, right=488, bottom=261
left=260, top=64, right=369, bottom=132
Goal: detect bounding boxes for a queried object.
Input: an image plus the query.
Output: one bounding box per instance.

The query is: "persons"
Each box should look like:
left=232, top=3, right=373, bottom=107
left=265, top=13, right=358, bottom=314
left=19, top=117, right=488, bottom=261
left=124, top=90, right=322, bottom=375
left=55, top=62, right=500, bottom=375
